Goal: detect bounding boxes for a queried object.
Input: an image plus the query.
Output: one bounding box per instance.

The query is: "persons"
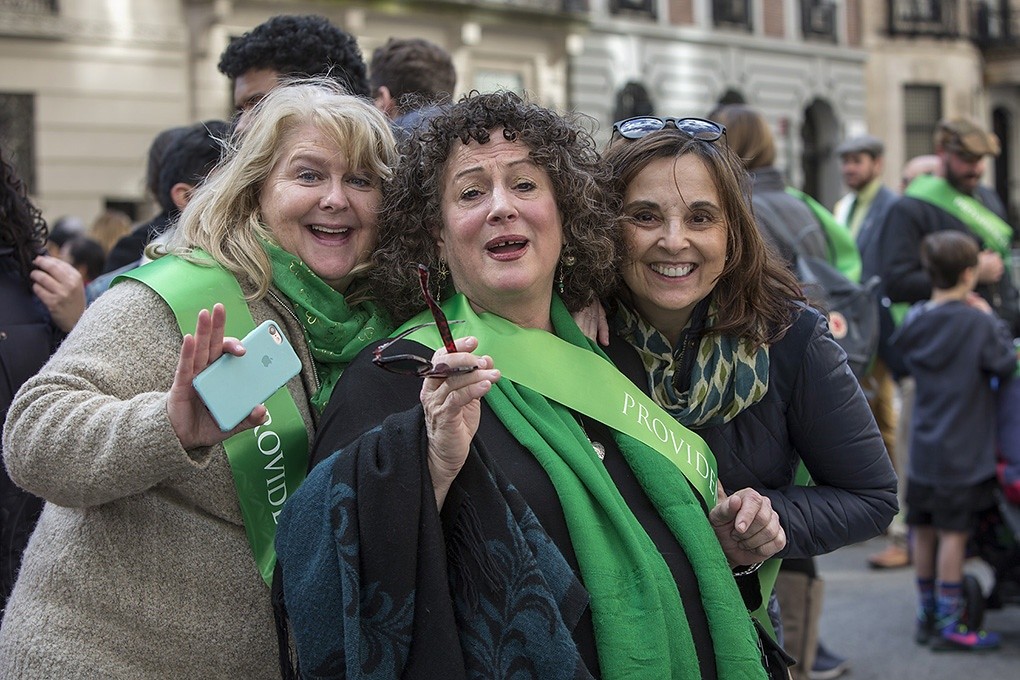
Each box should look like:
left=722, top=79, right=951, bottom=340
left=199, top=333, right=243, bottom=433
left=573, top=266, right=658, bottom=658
left=709, top=103, right=832, bottom=312
left=105, top=127, right=190, bottom=273
left=69, top=209, right=132, bottom=280
left=0, top=136, right=68, bottom=629
left=831, top=132, right=914, bottom=572
left=368, top=35, right=457, bottom=143
left=879, top=114, right=1020, bottom=561
left=47, top=214, right=84, bottom=261
left=277, top=79, right=798, bottom=680
left=156, top=120, right=239, bottom=220
left=570, top=116, right=899, bottom=646
left=31, top=14, right=372, bottom=333
left=900, top=154, right=945, bottom=193
left=0, top=48, right=399, bottom=680
left=886, top=230, right=1016, bottom=653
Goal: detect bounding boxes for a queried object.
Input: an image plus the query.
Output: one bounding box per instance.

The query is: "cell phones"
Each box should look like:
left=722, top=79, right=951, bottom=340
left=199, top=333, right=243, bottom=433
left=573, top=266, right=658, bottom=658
left=191, top=319, right=303, bottom=434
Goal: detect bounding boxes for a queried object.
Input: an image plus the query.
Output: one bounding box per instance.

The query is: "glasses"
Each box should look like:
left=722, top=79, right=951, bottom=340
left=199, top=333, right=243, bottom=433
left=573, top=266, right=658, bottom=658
left=372, top=262, right=478, bottom=379
left=608, top=116, right=729, bottom=162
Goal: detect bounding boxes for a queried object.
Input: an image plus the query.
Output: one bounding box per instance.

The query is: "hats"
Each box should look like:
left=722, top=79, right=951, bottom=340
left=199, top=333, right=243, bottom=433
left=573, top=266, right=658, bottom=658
left=936, top=120, right=1001, bottom=156
left=838, top=137, right=882, bottom=154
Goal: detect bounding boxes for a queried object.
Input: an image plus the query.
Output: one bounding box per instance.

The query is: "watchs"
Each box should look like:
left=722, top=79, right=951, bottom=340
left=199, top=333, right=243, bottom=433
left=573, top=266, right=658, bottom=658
left=734, top=562, right=757, bottom=576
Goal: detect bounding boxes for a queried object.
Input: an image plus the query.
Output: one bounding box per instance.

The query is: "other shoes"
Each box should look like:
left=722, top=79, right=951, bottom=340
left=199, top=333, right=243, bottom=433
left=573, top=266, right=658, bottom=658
left=917, top=615, right=935, bottom=643
left=931, top=623, right=999, bottom=652
left=870, top=547, right=912, bottom=568
left=806, top=644, right=846, bottom=680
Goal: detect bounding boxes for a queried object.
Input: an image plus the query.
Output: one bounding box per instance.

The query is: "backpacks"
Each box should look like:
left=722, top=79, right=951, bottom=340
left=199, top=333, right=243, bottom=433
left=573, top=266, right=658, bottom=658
left=752, top=197, right=879, bottom=376
